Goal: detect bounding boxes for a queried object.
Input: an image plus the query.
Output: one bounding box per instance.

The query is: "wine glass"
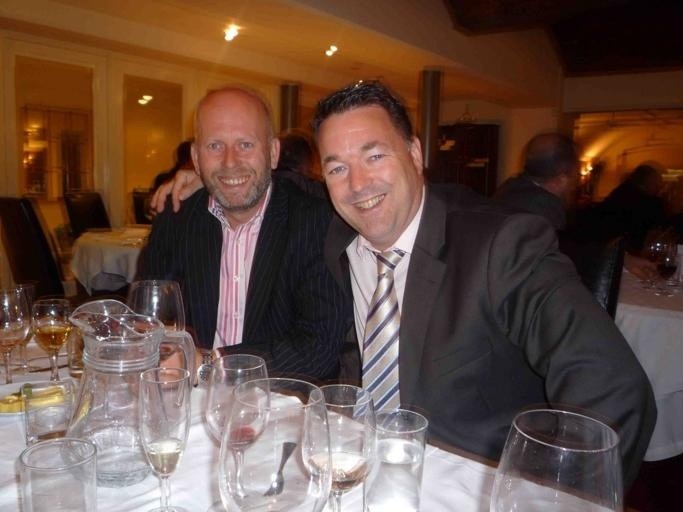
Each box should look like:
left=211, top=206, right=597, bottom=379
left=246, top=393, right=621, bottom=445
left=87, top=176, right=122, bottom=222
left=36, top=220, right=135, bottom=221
left=204, top=352, right=377, bottom=512
left=135, top=366, right=195, bottom=511
left=130, top=280, right=186, bottom=405
left=640, top=225, right=682, bottom=296
left=0, top=280, right=73, bottom=385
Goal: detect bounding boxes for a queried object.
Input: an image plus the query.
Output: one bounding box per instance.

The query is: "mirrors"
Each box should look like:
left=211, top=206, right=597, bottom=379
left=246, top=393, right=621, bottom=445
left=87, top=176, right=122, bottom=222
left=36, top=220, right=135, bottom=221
left=12, top=54, right=97, bottom=203
left=121, top=72, right=184, bottom=195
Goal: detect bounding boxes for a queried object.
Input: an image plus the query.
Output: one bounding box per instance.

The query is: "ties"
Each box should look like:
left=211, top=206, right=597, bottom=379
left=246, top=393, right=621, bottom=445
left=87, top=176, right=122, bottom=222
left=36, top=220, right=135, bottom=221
left=360, top=248, right=408, bottom=414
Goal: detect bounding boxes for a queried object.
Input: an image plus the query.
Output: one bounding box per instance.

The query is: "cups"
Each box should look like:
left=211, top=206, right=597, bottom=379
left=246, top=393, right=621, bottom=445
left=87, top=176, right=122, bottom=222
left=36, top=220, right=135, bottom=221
left=19, top=382, right=75, bottom=445
left=19, top=436, right=96, bottom=512
left=364, top=409, right=429, bottom=512
left=490, top=407, right=624, bottom=512
left=69, top=324, right=89, bottom=374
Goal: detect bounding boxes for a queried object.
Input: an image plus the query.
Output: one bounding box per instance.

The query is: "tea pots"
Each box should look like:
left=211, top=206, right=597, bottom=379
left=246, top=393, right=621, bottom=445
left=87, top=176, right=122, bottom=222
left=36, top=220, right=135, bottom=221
left=64, top=298, right=198, bottom=489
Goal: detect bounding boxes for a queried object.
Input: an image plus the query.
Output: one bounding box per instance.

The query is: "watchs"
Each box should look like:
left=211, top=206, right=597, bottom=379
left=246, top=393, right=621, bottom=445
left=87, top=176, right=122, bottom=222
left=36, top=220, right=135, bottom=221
left=197, top=350, right=221, bottom=386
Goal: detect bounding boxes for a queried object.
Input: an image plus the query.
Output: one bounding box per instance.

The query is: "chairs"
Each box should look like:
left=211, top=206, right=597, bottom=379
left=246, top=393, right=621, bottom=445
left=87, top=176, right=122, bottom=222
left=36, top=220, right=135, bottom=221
left=1, top=189, right=150, bottom=314
left=574, top=234, right=626, bottom=321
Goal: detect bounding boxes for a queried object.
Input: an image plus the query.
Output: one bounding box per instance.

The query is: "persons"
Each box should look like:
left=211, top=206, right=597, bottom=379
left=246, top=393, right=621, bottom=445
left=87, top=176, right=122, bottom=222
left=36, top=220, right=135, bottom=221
left=134, top=88, right=346, bottom=391
left=152, top=140, right=195, bottom=214
left=491, top=132, right=682, bottom=287
left=271, top=131, right=327, bottom=184
left=150, top=78, right=658, bottom=502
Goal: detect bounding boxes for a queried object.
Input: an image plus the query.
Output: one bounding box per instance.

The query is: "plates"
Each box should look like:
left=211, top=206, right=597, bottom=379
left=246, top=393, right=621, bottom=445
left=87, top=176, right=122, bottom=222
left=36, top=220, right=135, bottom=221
left=1, top=379, right=68, bottom=417
